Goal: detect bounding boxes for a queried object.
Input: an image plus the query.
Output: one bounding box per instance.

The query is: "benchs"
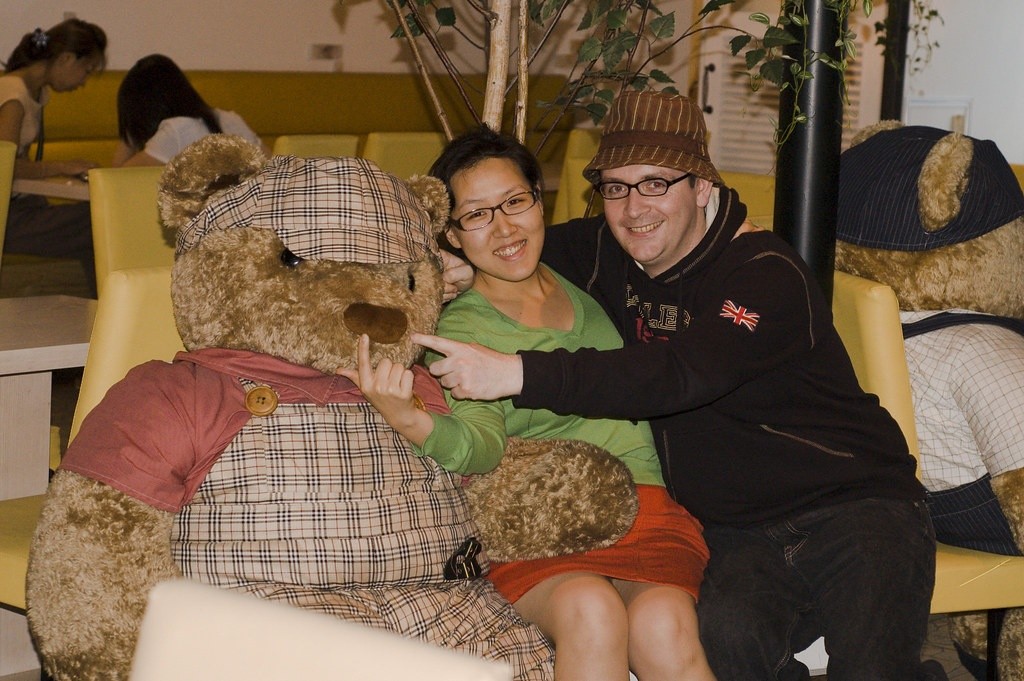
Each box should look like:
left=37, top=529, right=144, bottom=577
left=30, top=73, right=573, bottom=206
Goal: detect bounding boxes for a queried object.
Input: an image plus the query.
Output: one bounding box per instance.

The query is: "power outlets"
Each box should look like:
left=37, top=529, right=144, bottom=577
left=311, top=43, right=345, bottom=60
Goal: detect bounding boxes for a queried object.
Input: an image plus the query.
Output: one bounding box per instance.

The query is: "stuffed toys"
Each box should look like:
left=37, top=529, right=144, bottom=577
left=27, top=132, right=639, bottom=681
left=835, top=115, right=1024, bottom=681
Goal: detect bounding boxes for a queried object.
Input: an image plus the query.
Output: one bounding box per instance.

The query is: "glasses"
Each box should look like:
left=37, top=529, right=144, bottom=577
left=592, top=172, right=692, bottom=200
left=449, top=187, right=539, bottom=231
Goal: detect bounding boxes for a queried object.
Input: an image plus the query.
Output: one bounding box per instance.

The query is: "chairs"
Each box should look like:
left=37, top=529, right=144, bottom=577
left=0, top=120, right=774, bottom=614
left=130, top=578, right=515, bottom=681
left=830, top=270, right=1024, bottom=680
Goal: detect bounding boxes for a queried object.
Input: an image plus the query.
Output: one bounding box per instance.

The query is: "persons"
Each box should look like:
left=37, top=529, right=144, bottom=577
left=408, top=91, right=949, bottom=681
left=0, top=17, right=108, bottom=299
left=334, top=126, right=718, bottom=681
left=116, top=53, right=263, bottom=169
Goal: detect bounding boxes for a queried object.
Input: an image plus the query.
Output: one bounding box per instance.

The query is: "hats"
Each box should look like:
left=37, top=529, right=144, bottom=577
left=583, top=91, right=726, bottom=189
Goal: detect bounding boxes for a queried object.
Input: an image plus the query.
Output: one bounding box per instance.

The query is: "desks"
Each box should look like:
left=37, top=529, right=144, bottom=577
left=0, top=294, right=97, bottom=677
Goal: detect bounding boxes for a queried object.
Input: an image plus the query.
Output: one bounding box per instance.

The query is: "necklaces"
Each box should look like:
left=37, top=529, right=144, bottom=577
left=29, top=71, right=33, bottom=93
